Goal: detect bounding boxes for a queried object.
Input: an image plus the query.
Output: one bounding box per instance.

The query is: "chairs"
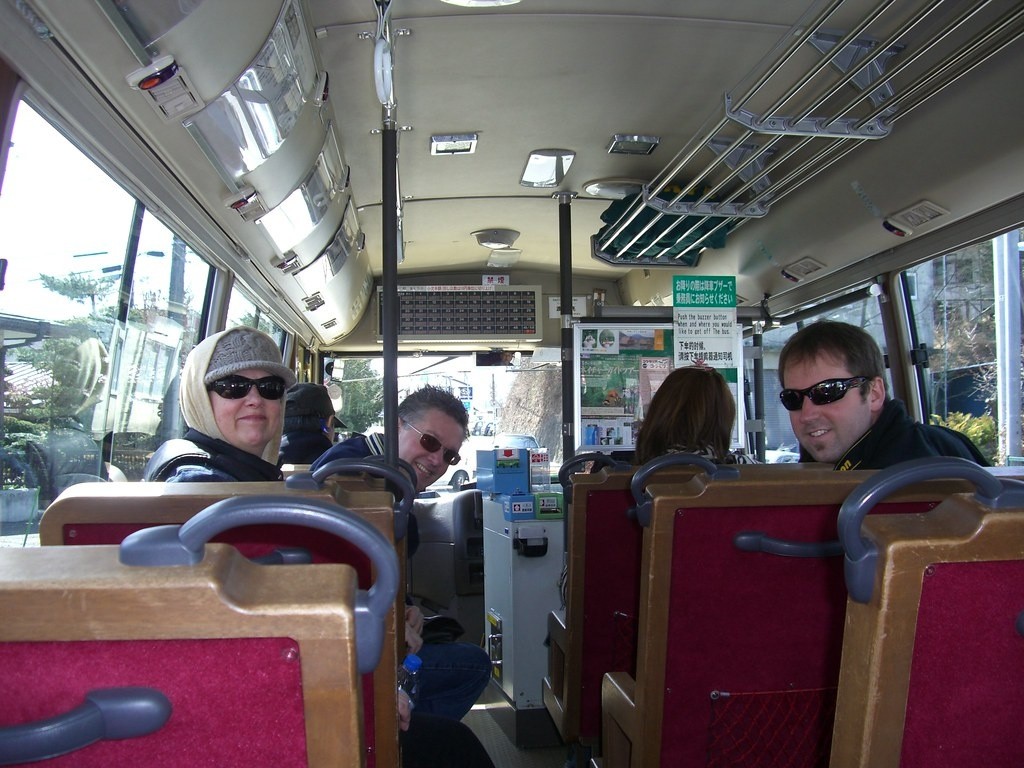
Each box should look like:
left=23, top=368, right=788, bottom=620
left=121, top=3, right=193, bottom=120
left=0, top=457, right=413, bottom=768
left=541, top=448, right=1024, bottom=768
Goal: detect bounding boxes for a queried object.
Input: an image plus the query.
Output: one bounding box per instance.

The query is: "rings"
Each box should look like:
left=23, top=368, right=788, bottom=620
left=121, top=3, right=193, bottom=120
left=408, top=699, right=412, bottom=706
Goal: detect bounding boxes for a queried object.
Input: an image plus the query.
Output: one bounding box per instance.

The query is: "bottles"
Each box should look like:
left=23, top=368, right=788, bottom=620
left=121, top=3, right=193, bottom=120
left=530, top=447, right=551, bottom=495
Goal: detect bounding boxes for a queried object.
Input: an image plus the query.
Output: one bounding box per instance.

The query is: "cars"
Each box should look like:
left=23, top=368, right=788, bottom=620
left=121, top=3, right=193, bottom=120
left=494, top=433, right=545, bottom=453
left=471, top=420, right=494, bottom=437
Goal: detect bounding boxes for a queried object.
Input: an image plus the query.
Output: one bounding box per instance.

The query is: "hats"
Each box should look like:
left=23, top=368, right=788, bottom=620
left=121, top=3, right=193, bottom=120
left=204, top=329, right=296, bottom=388
left=285, top=382, right=348, bottom=429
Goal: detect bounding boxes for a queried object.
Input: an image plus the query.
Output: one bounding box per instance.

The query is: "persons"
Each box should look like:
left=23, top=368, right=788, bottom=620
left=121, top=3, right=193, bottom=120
left=577, top=319, right=997, bottom=470
left=147, top=326, right=497, bottom=768
left=23, top=334, right=143, bottom=498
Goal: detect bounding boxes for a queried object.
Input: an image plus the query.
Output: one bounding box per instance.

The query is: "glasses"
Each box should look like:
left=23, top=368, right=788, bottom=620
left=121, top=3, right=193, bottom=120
left=779, top=376, right=874, bottom=412
left=399, top=417, right=461, bottom=466
left=206, top=374, right=287, bottom=401
